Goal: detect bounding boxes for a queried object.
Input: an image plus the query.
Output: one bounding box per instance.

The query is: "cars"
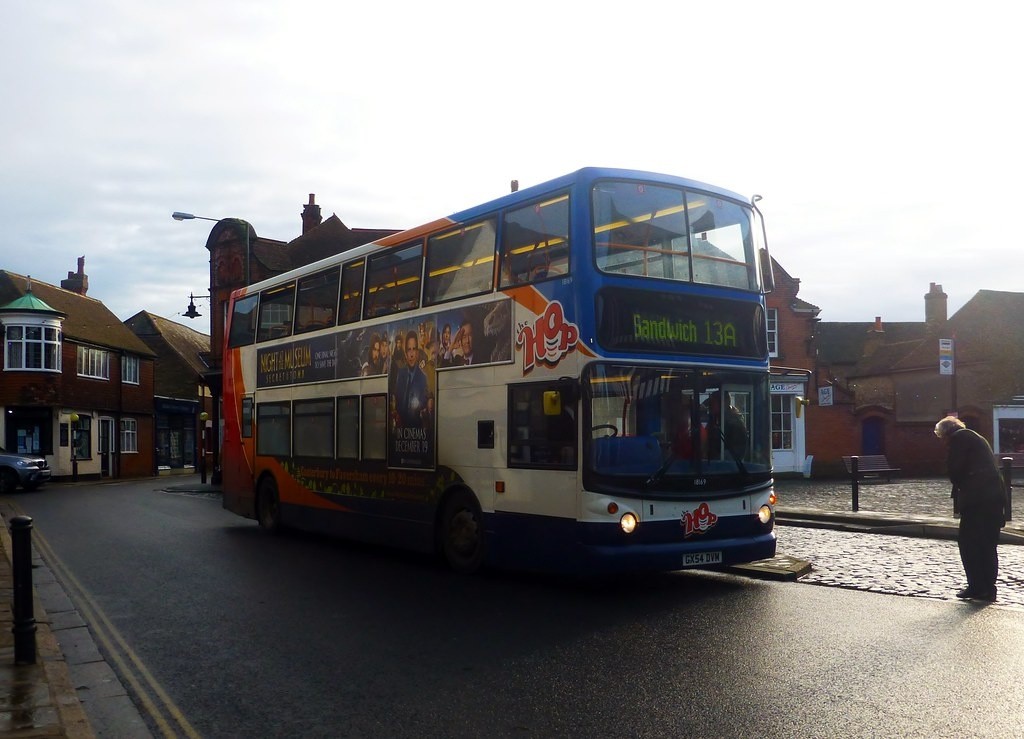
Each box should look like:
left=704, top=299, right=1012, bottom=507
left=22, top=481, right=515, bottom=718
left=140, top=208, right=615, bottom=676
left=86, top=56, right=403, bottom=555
left=0, top=445, right=52, bottom=495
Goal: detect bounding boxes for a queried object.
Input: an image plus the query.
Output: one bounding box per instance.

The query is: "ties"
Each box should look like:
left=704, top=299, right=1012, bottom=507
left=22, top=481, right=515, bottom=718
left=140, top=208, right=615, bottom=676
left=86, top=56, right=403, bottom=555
left=405, top=372, right=411, bottom=413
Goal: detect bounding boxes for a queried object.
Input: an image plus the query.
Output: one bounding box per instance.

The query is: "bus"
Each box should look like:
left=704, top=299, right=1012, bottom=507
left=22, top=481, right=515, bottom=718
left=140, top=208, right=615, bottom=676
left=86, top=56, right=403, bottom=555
left=219, top=169, right=813, bottom=584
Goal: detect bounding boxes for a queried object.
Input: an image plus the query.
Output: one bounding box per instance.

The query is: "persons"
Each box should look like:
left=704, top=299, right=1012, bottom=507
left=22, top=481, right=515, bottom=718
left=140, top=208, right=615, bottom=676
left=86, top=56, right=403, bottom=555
left=707, top=390, right=748, bottom=462
left=361, top=323, right=471, bottom=428
left=934, top=416, right=1007, bottom=601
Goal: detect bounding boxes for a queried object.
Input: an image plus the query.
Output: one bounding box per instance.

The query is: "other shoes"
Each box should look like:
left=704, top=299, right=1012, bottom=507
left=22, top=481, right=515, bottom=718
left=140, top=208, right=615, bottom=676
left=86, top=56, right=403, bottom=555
left=955, top=585, right=997, bottom=602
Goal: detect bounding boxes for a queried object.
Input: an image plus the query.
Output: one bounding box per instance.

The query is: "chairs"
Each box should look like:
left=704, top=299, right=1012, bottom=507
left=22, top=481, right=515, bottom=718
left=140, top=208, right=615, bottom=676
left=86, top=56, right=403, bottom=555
left=270, top=266, right=565, bottom=340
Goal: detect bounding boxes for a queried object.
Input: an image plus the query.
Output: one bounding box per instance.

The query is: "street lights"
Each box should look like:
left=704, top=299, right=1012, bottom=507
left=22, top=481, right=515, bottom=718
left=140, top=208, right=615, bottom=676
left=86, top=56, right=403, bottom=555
left=171, top=211, right=252, bottom=286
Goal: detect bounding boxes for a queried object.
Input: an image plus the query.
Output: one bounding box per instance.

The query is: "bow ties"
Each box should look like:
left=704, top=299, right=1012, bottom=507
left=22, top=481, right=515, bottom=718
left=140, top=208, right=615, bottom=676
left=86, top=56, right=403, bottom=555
left=459, top=357, right=470, bottom=366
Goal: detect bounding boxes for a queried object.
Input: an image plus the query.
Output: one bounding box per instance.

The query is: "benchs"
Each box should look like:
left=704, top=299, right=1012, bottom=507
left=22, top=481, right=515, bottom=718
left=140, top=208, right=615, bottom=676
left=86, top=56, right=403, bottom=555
left=993, top=453, right=1024, bottom=468
left=841, top=455, right=901, bottom=482
